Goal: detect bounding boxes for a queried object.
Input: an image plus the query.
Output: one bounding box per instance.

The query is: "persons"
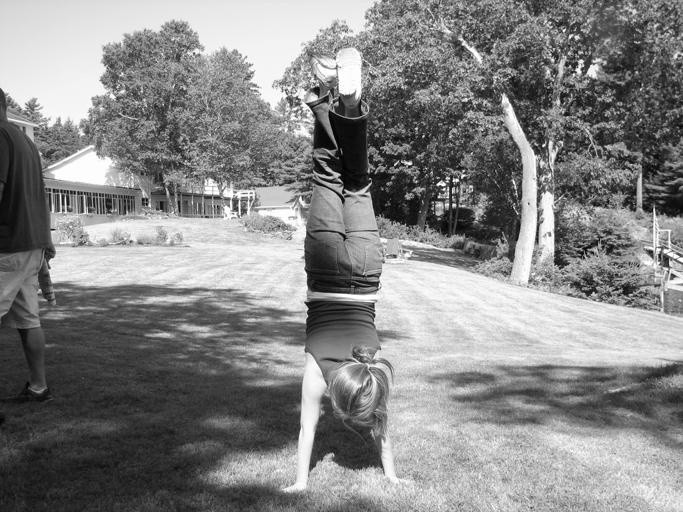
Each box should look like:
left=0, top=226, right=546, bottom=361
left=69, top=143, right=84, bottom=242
left=1, top=88, right=58, bottom=406
left=280, top=47, right=417, bottom=493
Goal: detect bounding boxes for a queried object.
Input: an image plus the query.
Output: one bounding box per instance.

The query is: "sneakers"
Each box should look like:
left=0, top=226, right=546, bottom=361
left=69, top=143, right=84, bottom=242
left=1, top=381, right=53, bottom=404
left=337, top=48, right=362, bottom=108
left=310, top=54, right=337, bottom=88
